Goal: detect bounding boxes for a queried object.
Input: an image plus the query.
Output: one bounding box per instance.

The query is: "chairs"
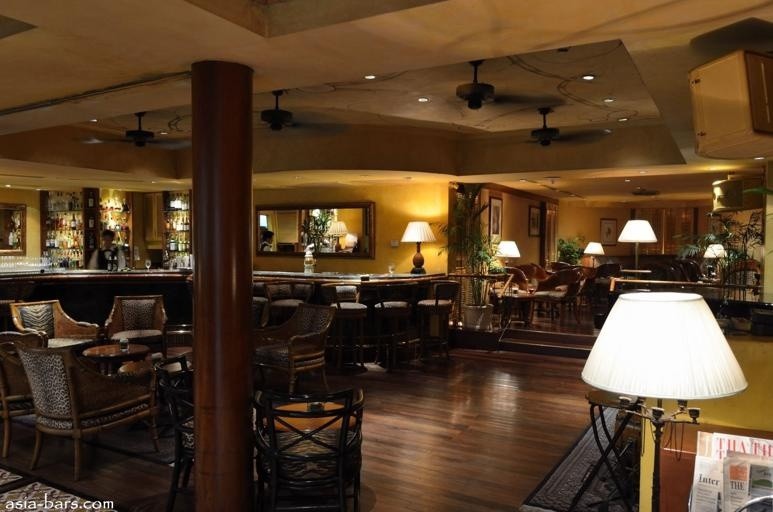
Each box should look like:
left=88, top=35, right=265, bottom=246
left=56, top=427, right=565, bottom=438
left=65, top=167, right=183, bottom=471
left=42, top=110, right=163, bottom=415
left=502, top=262, right=620, bottom=328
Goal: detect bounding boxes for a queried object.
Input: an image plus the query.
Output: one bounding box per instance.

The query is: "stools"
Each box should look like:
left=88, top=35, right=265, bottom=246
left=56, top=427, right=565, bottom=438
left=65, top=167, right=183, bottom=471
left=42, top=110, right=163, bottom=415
left=568, top=390, right=638, bottom=512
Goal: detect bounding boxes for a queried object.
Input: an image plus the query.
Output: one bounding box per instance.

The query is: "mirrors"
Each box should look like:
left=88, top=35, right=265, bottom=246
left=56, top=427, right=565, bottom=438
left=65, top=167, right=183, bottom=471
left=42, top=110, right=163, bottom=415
left=254, top=201, right=376, bottom=259
left=0, top=202, right=27, bottom=256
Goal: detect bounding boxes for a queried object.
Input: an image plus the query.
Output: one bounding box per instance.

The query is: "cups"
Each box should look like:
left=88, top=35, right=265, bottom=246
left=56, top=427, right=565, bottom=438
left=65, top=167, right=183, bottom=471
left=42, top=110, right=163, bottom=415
left=512, top=284, right=519, bottom=297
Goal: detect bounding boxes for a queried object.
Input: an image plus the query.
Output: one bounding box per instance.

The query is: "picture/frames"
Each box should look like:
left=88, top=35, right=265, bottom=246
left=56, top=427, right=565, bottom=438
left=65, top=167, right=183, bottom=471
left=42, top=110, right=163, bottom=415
left=489, top=196, right=502, bottom=246
left=599, top=217, right=617, bottom=246
left=528, top=205, right=541, bottom=237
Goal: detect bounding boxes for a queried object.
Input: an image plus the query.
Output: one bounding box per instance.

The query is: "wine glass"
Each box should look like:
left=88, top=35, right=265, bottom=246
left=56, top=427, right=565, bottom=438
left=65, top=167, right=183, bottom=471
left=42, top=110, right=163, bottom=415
left=389, top=266, right=396, bottom=275
left=1, top=256, right=52, bottom=273
left=528, top=284, right=537, bottom=294
left=145, top=259, right=152, bottom=270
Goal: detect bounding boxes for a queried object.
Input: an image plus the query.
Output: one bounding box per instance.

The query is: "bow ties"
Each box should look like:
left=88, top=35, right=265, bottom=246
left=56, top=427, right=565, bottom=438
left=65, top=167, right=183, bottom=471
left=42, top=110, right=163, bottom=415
left=104, top=249, right=113, bottom=251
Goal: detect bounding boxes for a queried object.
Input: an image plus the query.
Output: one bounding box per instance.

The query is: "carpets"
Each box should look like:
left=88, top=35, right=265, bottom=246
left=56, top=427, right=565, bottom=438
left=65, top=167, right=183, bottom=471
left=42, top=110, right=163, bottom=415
left=519, top=407, right=638, bottom=511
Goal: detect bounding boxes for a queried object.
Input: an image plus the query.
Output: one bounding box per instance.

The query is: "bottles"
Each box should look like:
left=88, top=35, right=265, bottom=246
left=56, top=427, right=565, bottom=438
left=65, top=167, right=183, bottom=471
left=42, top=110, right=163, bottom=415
left=119, top=338, right=129, bottom=353
left=107, top=256, right=113, bottom=272
left=8, top=229, right=21, bottom=250
left=99, top=194, right=130, bottom=249
left=163, top=190, right=192, bottom=270
left=43, top=191, right=84, bottom=269
left=304, top=249, right=314, bottom=274
left=113, top=255, right=118, bottom=272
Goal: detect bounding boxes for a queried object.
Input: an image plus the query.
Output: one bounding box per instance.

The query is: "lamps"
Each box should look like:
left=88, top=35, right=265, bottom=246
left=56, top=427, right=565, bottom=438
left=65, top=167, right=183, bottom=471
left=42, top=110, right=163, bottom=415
left=581, top=291, right=749, bottom=512
left=704, top=243, right=728, bottom=278
left=327, top=220, right=347, bottom=252
left=496, top=241, right=522, bottom=267
left=583, top=241, right=605, bottom=267
left=618, top=219, right=658, bottom=278
left=399, top=221, right=436, bottom=267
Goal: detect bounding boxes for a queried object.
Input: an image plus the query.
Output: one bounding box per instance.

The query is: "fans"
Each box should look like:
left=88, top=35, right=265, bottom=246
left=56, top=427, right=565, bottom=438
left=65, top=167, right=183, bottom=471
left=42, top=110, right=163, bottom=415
left=402, top=59, right=566, bottom=112
left=485, top=109, right=605, bottom=150
left=254, top=90, right=350, bottom=133
left=98, top=112, right=180, bottom=149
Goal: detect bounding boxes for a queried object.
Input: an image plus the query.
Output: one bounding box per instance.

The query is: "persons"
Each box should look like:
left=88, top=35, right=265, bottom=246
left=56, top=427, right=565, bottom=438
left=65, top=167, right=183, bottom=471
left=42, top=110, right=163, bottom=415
left=259, top=231, right=275, bottom=251
left=87, top=230, right=125, bottom=269
left=300, top=225, right=304, bottom=242
left=258, top=226, right=267, bottom=251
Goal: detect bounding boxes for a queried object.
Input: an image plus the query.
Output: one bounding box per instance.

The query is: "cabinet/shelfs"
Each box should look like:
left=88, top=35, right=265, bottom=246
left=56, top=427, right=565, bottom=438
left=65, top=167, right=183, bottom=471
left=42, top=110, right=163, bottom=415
left=162, top=191, right=191, bottom=253
left=44, top=191, right=84, bottom=267
left=99, top=190, right=127, bottom=249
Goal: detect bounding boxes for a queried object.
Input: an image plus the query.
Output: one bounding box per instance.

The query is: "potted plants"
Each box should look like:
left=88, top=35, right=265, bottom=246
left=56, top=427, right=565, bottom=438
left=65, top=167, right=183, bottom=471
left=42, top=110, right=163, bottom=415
left=439, top=201, right=494, bottom=330
